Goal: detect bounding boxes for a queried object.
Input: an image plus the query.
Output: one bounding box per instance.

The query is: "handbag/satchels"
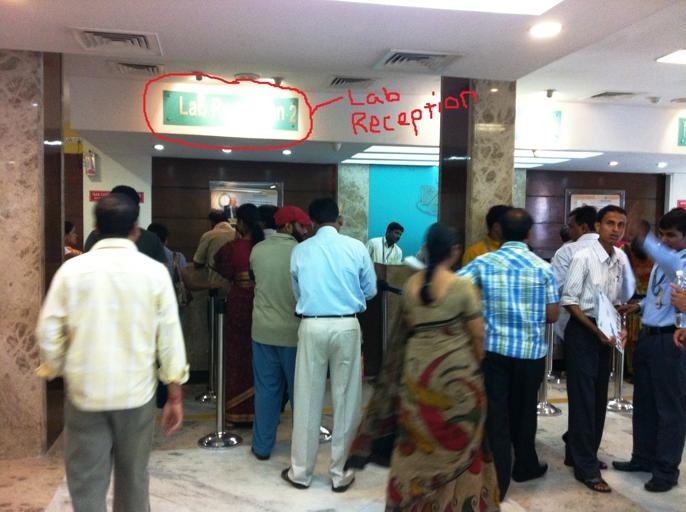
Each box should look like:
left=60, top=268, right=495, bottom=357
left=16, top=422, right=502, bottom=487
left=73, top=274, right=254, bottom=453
left=173, top=252, right=193, bottom=307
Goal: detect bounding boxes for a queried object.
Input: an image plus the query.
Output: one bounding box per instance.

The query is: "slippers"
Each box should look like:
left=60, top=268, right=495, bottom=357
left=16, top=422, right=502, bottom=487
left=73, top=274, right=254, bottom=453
left=564, top=459, right=607, bottom=469
left=574, top=471, right=611, bottom=492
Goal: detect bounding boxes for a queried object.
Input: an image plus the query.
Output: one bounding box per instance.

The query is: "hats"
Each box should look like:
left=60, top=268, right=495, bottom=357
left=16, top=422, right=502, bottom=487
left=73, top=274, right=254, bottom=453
left=274, top=206, right=312, bottom=226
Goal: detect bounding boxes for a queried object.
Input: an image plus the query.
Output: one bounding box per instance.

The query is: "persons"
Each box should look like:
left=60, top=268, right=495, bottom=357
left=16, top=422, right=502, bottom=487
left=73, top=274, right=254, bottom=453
left=193, top=211, right=243, bottom=303
left=214, top=203, right=264, bottom=429
left=35, top=194, right=191, bottom=512
left=669, top=283, right=686, bottom=349
left=548, top=207, right=636, bottom=342
left=559, top=204, right=627, bottom=493
left=559, top=224, right=572, bottom=244
left=248, top=206, right=312, bottom=461
left=64, top=220, right=81, bottom=256
left=84, top=185, right=166, bottom=264
left=612, top=207, right=686, bottom=492
left=342, top=222, right=501, bottom=512
left=461, top=205, right=513, bottom=267
left=147, top=223, right=188, bottom=284
left=281, top=198, right=378, bottom=493
left=256, top=200, right=279, bottom=239
left=455, top=208, right=560, bottom=503
left=364, top=222, right=404, bottom=265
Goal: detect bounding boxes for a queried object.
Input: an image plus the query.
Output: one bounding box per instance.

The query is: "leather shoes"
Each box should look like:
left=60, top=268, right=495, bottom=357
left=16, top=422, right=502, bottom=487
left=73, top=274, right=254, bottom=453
left=332, top=475, right=355, bottom=492
left=511, top=461, right=548, bottom=482
left=281, top=467, right=309, bottom=489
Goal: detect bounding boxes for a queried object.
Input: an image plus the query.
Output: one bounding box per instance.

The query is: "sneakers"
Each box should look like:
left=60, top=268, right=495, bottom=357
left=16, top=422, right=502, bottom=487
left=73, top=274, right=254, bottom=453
left=645, top=469, right=680, bottom=492
left=612, top=462, right=654, bottom=472
left=251, top=445, right=270, bottom=460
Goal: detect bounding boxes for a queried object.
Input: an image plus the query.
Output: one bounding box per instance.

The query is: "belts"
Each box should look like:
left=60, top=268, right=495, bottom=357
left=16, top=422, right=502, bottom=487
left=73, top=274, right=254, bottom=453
left=294, top=311, right=357, bottom=318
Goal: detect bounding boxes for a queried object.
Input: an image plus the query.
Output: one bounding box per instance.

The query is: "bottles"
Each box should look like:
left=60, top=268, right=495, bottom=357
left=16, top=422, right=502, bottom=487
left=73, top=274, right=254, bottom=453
left=672, top=270, right=686, bottom=329
